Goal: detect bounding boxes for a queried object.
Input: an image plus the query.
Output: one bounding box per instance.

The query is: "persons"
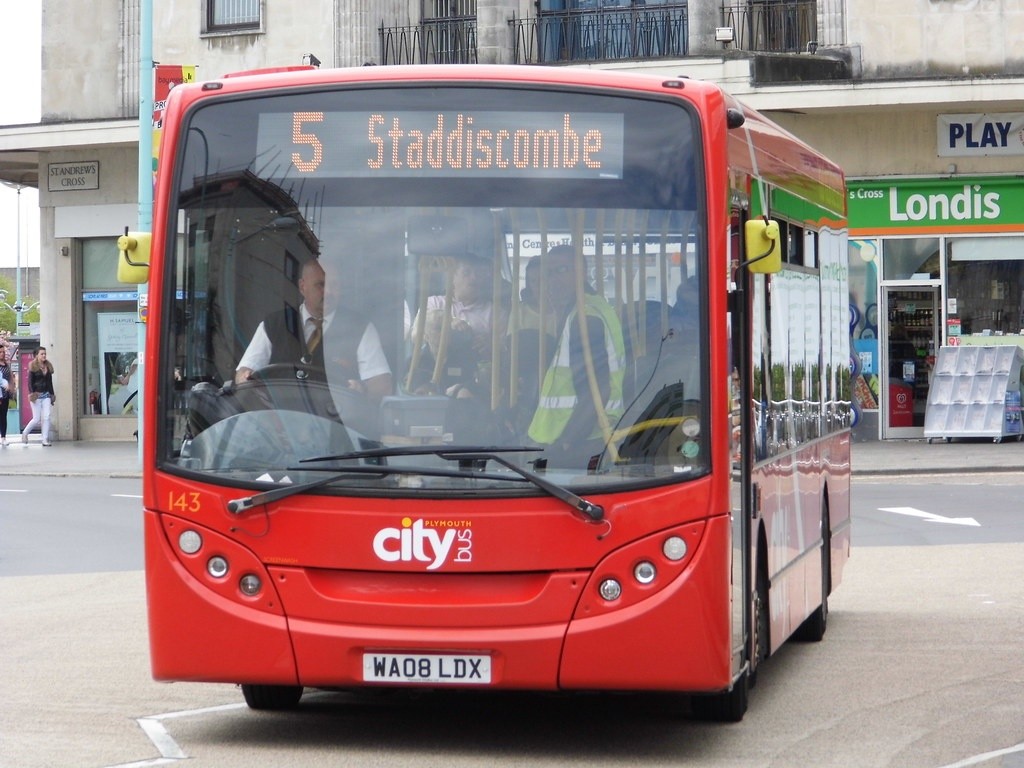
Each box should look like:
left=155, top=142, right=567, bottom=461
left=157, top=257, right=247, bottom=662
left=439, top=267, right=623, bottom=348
left=404, top=243, right=701, bottom=469
left=105, top=352, right=138, bottom=415
left=22, top=347, right=56, bottom=446
left=0, top=345, right=14, bottom=446
left=235, top=253, right=393, bottom=397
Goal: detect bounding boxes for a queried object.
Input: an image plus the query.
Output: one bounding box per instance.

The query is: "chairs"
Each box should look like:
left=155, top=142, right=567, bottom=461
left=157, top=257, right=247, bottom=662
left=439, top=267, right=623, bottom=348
left=621, top=299, right=673, bottom=364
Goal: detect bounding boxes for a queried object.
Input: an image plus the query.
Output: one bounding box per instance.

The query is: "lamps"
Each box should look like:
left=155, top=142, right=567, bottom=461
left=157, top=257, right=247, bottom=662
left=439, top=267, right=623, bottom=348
left=715, top=26, right=735, bottom=44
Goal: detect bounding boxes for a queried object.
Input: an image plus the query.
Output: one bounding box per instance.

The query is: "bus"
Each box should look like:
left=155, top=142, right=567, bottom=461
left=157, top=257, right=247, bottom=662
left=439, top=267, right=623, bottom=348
left=114, top=60, right=855, bottom=720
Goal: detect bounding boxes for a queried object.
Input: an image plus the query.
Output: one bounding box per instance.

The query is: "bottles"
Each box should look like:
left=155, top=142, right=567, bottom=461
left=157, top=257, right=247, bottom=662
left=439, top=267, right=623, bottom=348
left=896, top=291, right=933, bottom=301
left=888, top=310, right=942, bottom=327
left=911, top=331, right=934, bottom=358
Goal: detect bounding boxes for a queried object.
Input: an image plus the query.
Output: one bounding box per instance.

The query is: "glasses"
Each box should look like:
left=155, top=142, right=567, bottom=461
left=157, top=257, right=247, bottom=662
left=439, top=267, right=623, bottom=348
left=547, top=264, right=575, bottom=278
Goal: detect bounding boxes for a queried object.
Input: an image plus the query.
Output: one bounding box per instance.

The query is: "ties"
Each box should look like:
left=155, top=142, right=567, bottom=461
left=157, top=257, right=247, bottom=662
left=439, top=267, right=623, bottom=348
left=307, top=318, right=325, bottom=354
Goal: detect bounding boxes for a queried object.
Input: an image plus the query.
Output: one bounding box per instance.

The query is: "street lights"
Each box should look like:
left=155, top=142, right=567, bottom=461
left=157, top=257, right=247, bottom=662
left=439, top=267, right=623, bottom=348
left=0, top=289, right=41, bottom=334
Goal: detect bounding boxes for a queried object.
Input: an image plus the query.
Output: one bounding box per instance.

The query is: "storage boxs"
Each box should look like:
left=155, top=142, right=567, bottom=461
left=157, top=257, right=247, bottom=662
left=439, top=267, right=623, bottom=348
left=972, top=328, right=1024, bottom=336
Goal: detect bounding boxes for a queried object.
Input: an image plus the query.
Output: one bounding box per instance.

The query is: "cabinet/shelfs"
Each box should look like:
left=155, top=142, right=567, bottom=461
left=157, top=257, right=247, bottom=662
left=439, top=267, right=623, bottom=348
left=888, top=300, right=941, bottom=388
left=923, top=344, right=1024, bottom=443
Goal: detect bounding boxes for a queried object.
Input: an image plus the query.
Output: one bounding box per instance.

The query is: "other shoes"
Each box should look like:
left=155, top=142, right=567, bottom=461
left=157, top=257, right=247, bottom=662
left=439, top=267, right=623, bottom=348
left=42, top=441, right=51, bottom=446
left=0, top=438, right=8, bottom=445
left=22, top=434, right=28, bottom=443
